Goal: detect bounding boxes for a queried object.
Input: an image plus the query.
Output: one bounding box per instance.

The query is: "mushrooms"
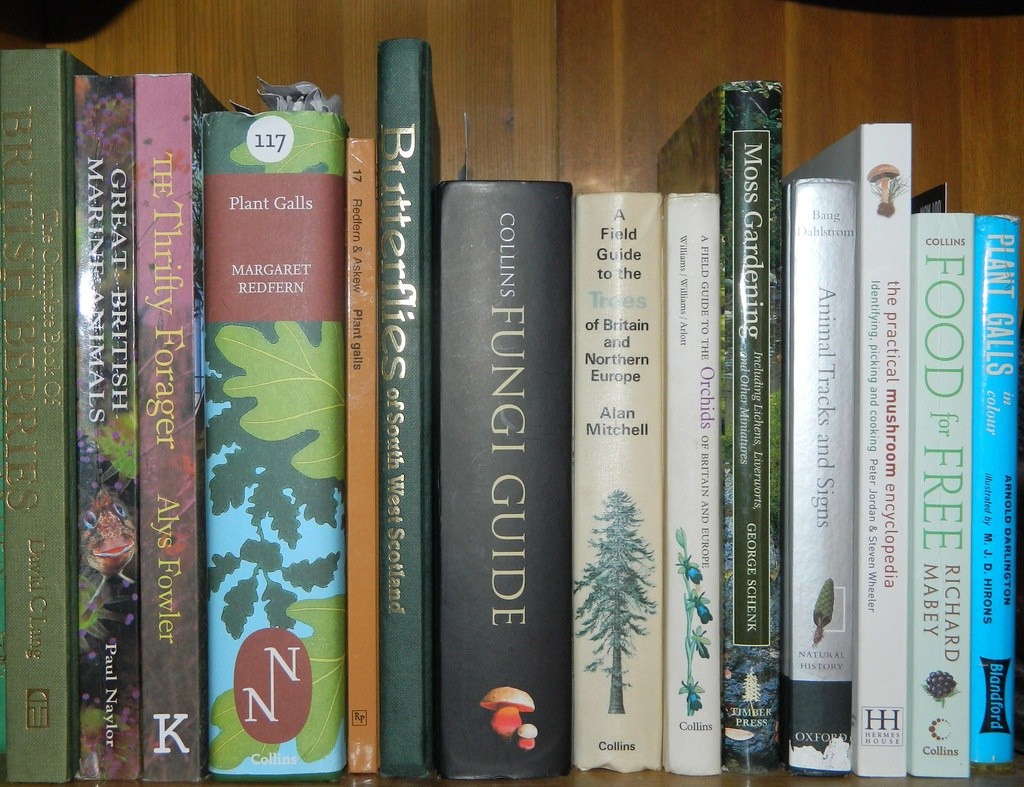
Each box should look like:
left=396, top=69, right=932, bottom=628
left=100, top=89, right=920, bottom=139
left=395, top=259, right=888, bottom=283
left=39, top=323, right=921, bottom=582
left=480, top=687, right=538, bottom=751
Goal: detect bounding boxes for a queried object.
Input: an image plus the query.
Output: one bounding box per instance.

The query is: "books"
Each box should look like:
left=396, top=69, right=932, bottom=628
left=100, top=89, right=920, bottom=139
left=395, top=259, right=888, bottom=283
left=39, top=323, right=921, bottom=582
left=1, top=39, right=1024, bottom=786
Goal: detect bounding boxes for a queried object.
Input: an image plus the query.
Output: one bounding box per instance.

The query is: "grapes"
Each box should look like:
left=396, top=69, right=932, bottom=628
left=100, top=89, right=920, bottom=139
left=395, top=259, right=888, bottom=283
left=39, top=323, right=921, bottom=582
left=925, top=671, right=957, bottom=698
